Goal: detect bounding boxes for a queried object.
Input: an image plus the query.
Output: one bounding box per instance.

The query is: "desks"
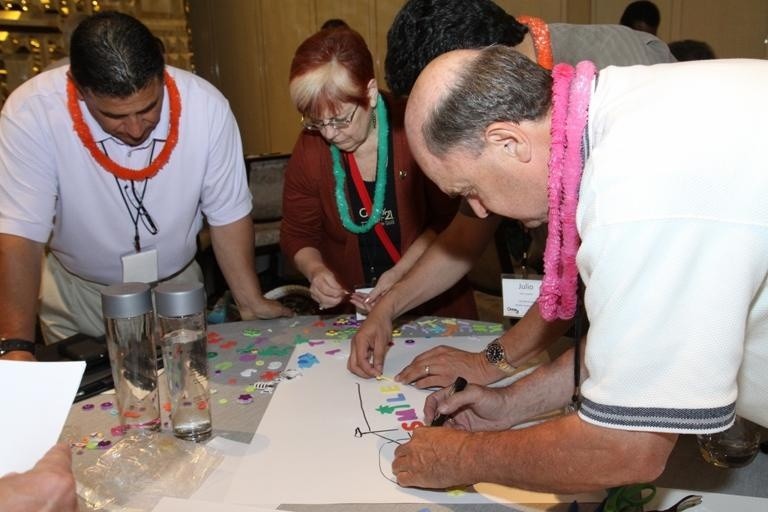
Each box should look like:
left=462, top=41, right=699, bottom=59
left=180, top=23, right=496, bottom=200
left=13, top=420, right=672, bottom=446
left=59, top=313, right=767, bottom=511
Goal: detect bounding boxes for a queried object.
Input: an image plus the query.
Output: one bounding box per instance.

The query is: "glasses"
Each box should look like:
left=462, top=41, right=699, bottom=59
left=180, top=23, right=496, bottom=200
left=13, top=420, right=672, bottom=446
left=301, top=103, right=359, bottom=131
left=125, top=184, right=159, bottom=235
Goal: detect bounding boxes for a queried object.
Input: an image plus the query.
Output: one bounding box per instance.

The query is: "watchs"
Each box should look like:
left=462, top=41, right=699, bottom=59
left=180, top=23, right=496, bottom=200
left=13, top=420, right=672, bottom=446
left=486, top=338, right=519, bottom=378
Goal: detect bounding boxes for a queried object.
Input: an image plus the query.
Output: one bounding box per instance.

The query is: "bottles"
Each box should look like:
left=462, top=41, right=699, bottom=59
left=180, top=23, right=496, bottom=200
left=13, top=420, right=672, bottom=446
left=152, top=279, right=212, bottom=443
left=93, top=280, right=163, bottom=437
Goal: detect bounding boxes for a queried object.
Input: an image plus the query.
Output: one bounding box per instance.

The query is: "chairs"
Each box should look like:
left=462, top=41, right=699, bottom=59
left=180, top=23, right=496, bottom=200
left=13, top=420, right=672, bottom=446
left=195, top=153, right=292, bottom=296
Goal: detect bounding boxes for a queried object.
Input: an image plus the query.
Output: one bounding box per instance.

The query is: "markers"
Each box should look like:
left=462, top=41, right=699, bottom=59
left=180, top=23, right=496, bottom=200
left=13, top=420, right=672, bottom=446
left=430, top=377, right=467, bottom=427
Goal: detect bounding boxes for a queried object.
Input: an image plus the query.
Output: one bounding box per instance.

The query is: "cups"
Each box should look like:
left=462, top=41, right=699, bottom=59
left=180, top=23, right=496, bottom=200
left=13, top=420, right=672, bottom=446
left=693, top=415, right=762, bottom=470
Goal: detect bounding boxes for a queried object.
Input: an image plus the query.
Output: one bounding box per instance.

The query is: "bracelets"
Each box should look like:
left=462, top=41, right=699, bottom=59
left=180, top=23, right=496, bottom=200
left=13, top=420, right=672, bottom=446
left=0, top=339, right=36, bottom=355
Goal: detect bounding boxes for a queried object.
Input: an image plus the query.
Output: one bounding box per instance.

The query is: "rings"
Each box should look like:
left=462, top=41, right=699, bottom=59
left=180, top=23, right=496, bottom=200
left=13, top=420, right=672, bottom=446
left=425, top=366, right=430, bottom=375
left=318, top=303, right=324, bottom=311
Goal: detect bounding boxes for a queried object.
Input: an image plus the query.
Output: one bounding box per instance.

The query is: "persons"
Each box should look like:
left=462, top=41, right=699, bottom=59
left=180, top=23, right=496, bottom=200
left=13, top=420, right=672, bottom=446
left=391, top=41, right=768, bottom=489
left=621, top=1, right=660, bottom=36
left=669, top=38, right=716, bottom=60
left=348, top=0, right=676, bottom=389
left=0, top=441, right=78, bottom=512
left=0, top=10, right=297, bottom=361
left=277, top=19, right=478, bottom=321
